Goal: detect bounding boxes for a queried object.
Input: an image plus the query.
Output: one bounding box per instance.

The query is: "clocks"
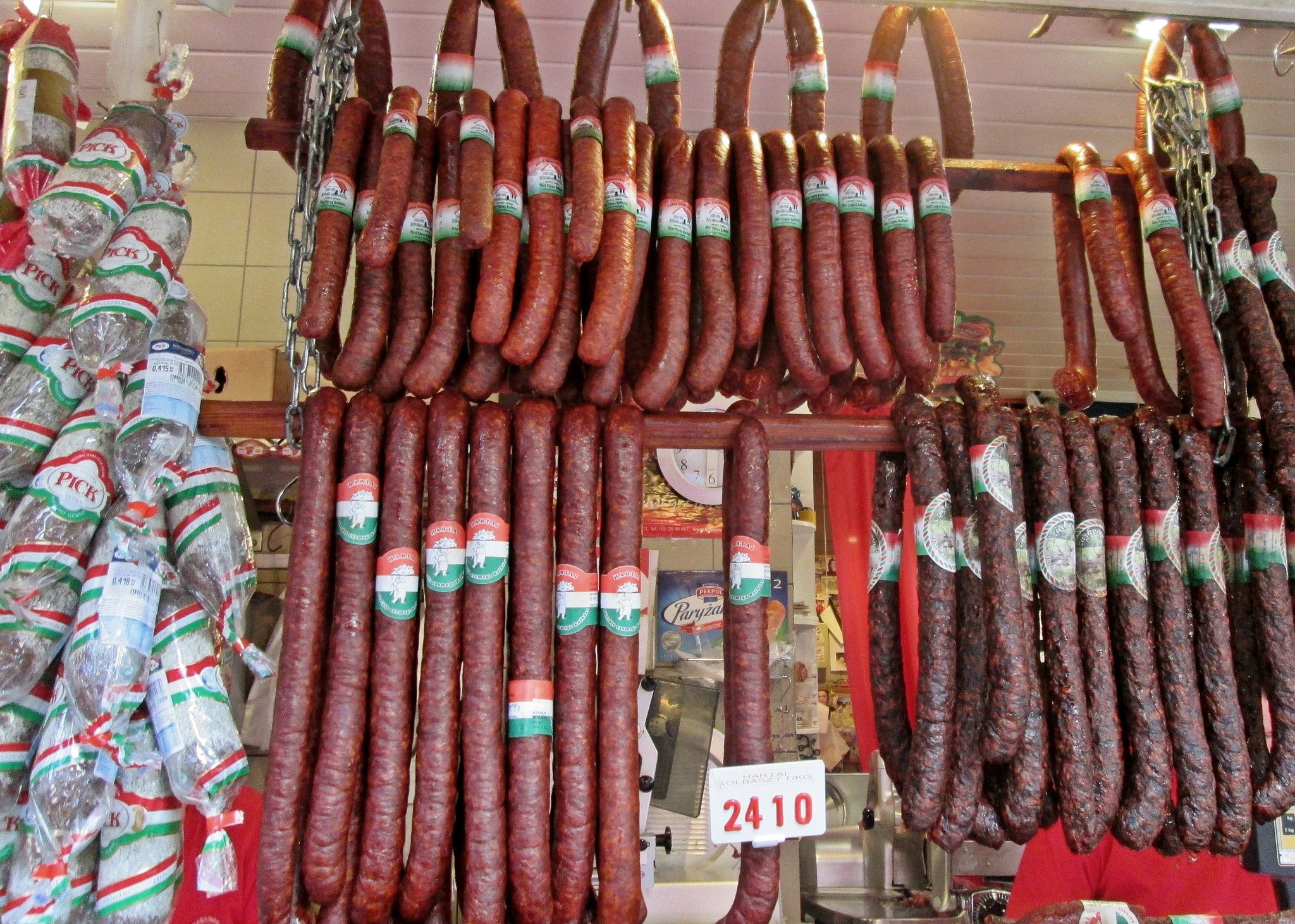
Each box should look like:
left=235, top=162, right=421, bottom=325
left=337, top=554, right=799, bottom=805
left=655, top=391, right=729, bottom=507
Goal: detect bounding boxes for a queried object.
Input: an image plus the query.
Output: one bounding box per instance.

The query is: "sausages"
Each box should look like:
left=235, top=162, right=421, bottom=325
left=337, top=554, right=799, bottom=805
left=0, top=0, right=1295, bottom=924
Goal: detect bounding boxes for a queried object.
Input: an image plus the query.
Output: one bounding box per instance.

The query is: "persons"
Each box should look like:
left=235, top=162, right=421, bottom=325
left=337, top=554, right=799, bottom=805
left=1003, top=767, right=1278, bottom=920
left=167, top=783, right=262, bottom=924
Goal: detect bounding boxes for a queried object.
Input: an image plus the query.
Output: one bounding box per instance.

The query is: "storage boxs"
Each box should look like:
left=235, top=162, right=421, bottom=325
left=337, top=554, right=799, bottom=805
left=201, top=342, right=301, bottom=404
